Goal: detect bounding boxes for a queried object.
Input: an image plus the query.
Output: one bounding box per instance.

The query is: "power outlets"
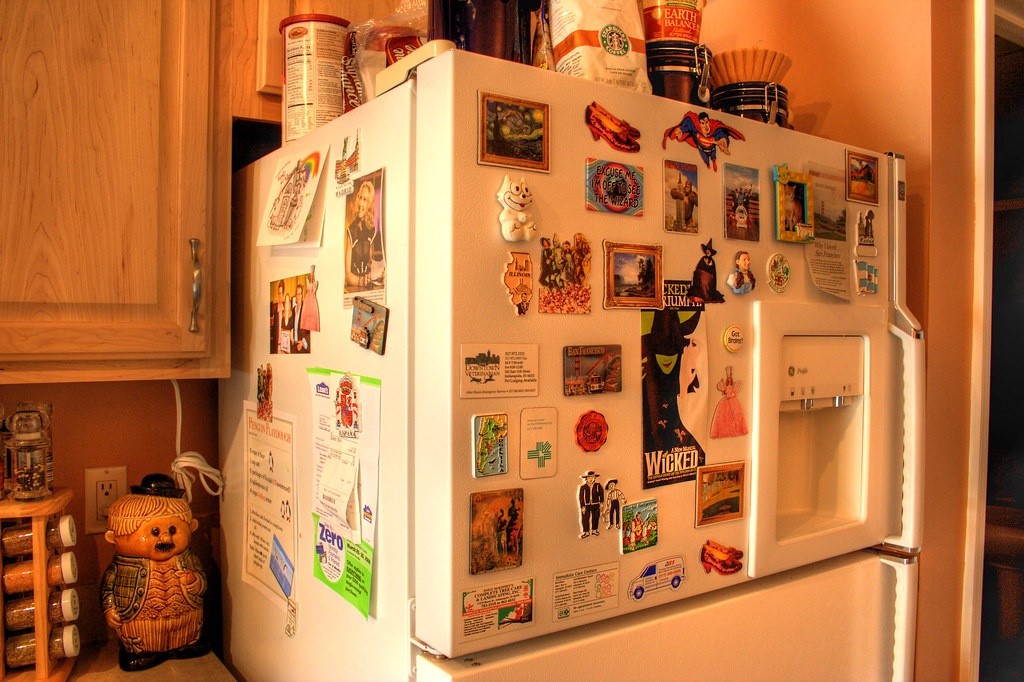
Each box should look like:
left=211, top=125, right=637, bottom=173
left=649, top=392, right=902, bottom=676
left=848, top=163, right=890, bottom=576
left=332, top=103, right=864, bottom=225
left=84, top=465, right=130, bottom=536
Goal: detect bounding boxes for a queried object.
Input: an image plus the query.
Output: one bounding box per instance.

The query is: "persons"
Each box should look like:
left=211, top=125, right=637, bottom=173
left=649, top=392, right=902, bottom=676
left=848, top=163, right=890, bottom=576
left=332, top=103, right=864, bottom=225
left=345, top=180, right=385, bottom=287
left=272, top=280, right=310, bottom=355
left=99, top=473, right=212, bottom=672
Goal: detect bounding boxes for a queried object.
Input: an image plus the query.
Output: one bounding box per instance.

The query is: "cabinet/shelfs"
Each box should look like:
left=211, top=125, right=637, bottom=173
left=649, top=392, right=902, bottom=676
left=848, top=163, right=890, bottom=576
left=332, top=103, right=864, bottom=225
left=0, top=0, right=440, bottom=681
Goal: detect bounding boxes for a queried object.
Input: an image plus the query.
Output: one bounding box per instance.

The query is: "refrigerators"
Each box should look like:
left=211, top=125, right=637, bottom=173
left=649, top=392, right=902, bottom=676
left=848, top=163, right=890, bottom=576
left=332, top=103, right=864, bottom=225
left=215, top=39, right=927, bottom=682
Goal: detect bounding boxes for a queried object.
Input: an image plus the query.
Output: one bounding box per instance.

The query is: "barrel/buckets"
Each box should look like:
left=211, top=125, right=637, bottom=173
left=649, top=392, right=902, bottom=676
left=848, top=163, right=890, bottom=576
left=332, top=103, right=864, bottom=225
left=708, top=82, right=788, bottom=129
left=646, top=41, right=712, bottom=108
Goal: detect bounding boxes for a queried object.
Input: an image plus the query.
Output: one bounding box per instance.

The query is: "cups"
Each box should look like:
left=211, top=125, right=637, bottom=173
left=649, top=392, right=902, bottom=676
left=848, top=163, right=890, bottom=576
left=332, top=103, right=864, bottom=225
left=6, top=624, right=79, bottom=668
left=2, top=515, right=77, bottom=556
left=5, top=589, right=79, bottom=630
left=17, top=401, right=53, bottom=491
left=3, top=552, right=78, bottom=594
